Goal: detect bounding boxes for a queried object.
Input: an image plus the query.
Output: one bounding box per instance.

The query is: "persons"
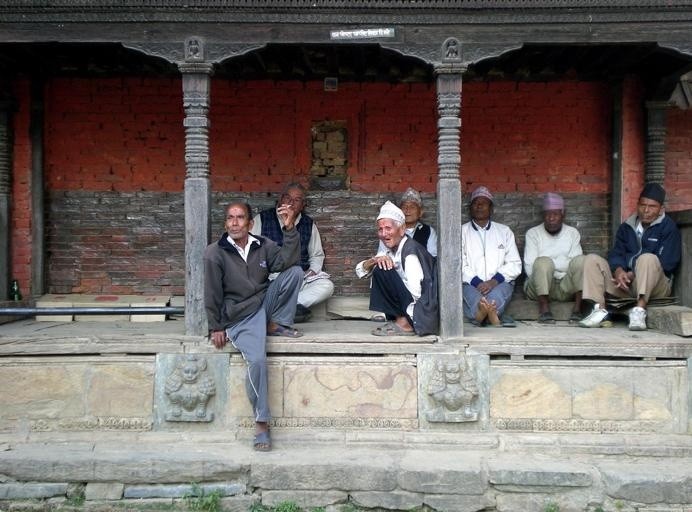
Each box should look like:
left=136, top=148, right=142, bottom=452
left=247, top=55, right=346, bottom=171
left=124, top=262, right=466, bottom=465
left=462, top=186, right=523, bottom=328
left=248, top=182, right=334, bottom=323
left=524, top=193, right=589, bottom=325
left=578, top=183, right=682, bottom=331
left=203, top=203, right=304, bottom=452
left=400, top=185, right=438, bottom=258
left=355, top=199, right=440, bottom=336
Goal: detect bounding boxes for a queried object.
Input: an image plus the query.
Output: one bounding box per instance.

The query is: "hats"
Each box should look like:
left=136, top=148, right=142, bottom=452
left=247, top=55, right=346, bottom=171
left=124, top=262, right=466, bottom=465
left=374, top=199, right=406, bottom=228
left=469, top=185, right=495, bottom=208
left=639, top=178, right=666, bottom=207
left=399, top=185, right=422, bottom=210
left=537, top=189, right=567, bottom=215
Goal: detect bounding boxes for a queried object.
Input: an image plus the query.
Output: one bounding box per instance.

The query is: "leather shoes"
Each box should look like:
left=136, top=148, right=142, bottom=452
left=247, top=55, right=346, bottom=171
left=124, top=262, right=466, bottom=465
left=294, top=303, right=313, bottom=323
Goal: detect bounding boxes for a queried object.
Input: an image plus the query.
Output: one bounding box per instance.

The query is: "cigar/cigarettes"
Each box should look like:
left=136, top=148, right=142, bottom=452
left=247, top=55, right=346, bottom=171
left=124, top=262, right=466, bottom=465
left=287, top=205, right=292, bottom=208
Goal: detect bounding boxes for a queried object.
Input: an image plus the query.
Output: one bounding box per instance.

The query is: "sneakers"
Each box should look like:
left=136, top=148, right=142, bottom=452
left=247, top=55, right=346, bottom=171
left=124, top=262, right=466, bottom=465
left=576, top=299, right=617, bottom=332
left=627, top=306, right=647, bottom=335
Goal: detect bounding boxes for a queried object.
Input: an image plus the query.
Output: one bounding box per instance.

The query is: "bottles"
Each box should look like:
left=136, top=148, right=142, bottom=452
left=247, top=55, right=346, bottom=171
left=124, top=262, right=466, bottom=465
left=11, top=279, right=20, bottom=302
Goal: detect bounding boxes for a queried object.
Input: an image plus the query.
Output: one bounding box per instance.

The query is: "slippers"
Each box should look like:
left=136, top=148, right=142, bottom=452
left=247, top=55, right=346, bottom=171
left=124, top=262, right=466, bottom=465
left=470, top=317, right=485, bottom=327
left=538, top=311, right=557, bottom=329
left=370, top=321, right=417, bottom=338
left=251, top=423, right=275, bottom=456
left=501, top=313, right=516, bottom=328
left=569, top=310, right=582, bottom=325
left=266, top=323, right=305, bottom=340
